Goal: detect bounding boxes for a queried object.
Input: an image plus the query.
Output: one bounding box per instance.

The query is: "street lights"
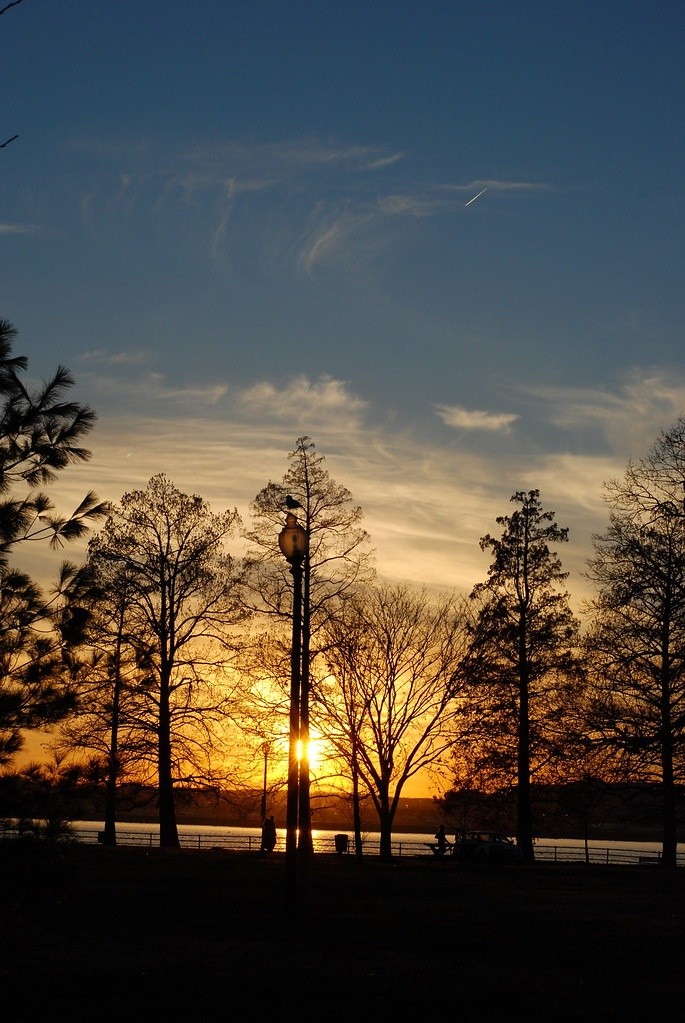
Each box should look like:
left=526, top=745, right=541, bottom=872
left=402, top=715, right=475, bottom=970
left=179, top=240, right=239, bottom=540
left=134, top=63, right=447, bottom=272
left=279, top=512, right=317, bottom=887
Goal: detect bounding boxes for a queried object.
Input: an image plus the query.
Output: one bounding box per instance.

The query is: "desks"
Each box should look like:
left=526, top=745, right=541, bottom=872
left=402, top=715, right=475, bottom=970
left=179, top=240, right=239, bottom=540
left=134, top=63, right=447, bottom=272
left=424, top=843, right=450, bottom=866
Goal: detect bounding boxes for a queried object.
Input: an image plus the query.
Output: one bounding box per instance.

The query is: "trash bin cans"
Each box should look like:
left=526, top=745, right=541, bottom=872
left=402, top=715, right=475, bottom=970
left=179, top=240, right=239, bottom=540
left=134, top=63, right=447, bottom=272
left=335, top=835, right=348, bottom=855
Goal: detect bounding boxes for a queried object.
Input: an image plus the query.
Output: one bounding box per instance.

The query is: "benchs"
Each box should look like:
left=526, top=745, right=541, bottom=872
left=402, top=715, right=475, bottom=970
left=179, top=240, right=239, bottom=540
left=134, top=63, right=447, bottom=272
left=418, top=854, right=454, bottom=867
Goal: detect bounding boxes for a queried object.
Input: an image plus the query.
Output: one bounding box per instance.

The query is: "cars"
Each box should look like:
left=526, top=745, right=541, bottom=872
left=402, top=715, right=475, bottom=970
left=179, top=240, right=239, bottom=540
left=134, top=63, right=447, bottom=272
left=458, top=830, right=524, bottom=873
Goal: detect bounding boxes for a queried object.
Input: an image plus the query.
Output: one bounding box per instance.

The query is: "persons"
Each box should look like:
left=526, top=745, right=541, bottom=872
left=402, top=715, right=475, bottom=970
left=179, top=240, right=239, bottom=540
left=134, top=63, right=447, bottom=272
left=263, top=816, right=276, bottom=852
left=435, top=824, right=450, bottom=856
